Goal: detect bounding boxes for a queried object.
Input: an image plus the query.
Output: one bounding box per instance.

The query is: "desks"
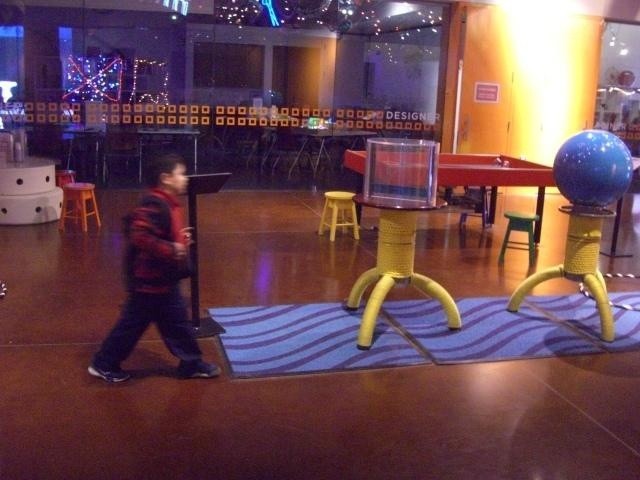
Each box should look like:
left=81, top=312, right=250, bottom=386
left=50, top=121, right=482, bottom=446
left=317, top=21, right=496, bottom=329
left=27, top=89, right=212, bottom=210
left=60, top=123, right=200, bottom=184
left=341, top=146, right=559, bottom=244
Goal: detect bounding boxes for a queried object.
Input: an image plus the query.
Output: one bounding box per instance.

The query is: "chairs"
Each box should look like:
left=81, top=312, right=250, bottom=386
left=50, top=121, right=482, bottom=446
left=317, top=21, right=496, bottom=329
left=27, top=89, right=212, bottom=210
left=102, top=122, right=143, bottom=185
left=252, top=124, right=378, bottom=184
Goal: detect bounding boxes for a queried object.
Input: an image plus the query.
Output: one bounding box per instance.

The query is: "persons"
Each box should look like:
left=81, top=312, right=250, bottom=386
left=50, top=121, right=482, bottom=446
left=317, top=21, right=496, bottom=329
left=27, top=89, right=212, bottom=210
left=87, top=155, right=222, bottom=382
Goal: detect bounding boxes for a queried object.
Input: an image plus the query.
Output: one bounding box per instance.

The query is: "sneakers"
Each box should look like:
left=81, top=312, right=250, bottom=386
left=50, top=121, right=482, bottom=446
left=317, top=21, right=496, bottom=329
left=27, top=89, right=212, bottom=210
left=87, top=359, right=132, bottom=383
left=175, top=359, right=222, bottom=379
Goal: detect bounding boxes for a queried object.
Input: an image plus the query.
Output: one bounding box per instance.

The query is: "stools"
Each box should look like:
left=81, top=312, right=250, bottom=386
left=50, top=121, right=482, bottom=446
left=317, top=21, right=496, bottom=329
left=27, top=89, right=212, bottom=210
left=55, top=170, right=100, bottom=232
left=318, top=186, right=541, bottom=264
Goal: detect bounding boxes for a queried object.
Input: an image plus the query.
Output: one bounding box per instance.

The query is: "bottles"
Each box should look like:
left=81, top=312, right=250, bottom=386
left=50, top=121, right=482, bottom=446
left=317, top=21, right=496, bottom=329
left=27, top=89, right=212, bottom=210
left=8, top=133, right=23, bottom=162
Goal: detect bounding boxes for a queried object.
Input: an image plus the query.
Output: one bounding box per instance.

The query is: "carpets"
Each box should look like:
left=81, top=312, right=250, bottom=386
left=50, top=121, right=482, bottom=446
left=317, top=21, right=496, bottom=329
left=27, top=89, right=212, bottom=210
left=207, top=300, right=432, bottom=379
left=517, top=291, right=640, bottom=355
left=380, top=296, right=608, bottom=366
